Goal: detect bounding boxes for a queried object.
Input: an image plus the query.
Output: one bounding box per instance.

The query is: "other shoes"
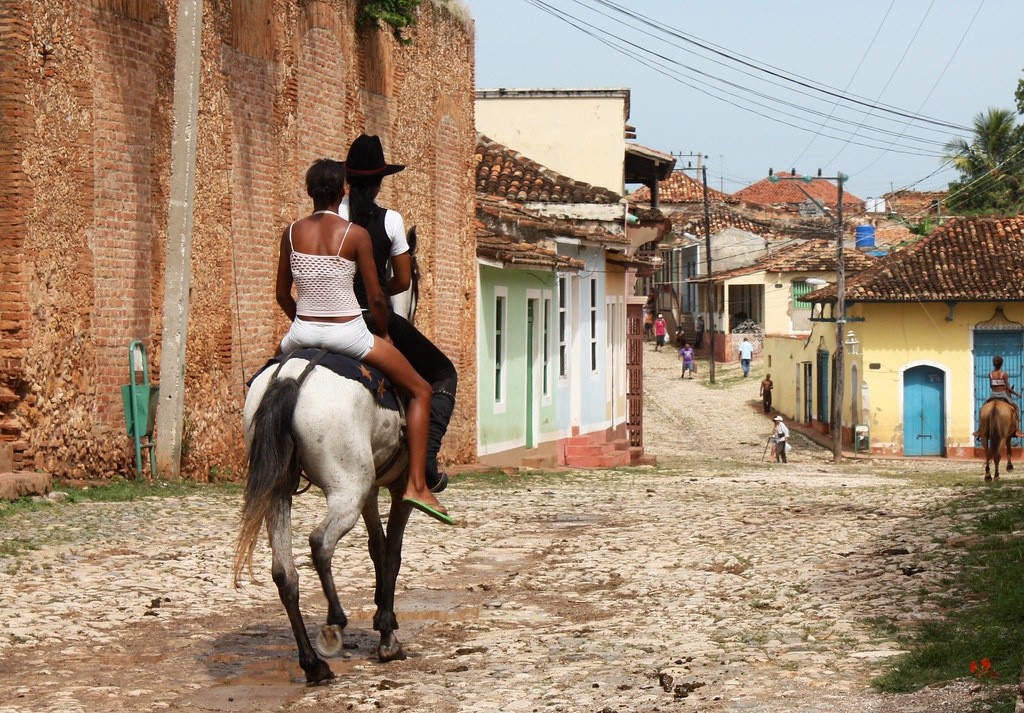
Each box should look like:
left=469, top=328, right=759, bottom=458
left=1015, top=431, right=1024, bottom=437
left=973, top=431, right=981, bottom=436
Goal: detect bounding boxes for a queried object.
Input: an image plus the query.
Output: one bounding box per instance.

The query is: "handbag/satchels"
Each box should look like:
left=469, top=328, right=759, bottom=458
left=693, top=361, right=697, bottom=373
left=664, top=333, right=669, bottom=342
left=777, top=429, right=784, bottom=438
left=785, top=442, right=791, bottom=451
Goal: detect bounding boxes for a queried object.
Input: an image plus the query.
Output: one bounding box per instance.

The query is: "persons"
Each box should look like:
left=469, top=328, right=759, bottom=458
left=738, top=337, right=753, bottom=376
left=678, top=343, right=695, bottom=379
left=759, top=374, right=774, bottom=412
left=675, top=326, right=685, bottom=346
left=694, top=315, right=704, bottom=348
left=643, top=308, right=653, bottom=341
left=653, top=313, right=666, bottom=353
left=275, top=158, right=454, bottom=525
left=972, top=356, right=1024, bottom=438
left=769, top=415, right=791, bottom=463
left=337, top=134, right=458, bottom=493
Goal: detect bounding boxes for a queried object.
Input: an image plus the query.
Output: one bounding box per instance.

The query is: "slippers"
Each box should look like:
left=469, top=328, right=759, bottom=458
left=402, top=498, right=454, bottom=525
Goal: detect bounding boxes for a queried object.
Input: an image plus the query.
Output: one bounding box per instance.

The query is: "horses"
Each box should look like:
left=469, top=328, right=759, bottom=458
left=970, top=385, right=1018, bottom=483
left=234, top=219, right=423, bottom=691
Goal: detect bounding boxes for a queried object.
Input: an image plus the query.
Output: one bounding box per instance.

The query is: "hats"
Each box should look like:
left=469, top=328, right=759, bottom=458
left=335, top=134, right=405, bottom=179
left=774, top=416, right=783, bottom=421
left=658, top=314, right=662, bottom=317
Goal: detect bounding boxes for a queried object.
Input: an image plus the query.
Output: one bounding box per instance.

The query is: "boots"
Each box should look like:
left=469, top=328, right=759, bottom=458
left=426, top=390, right=454, bottom=492
